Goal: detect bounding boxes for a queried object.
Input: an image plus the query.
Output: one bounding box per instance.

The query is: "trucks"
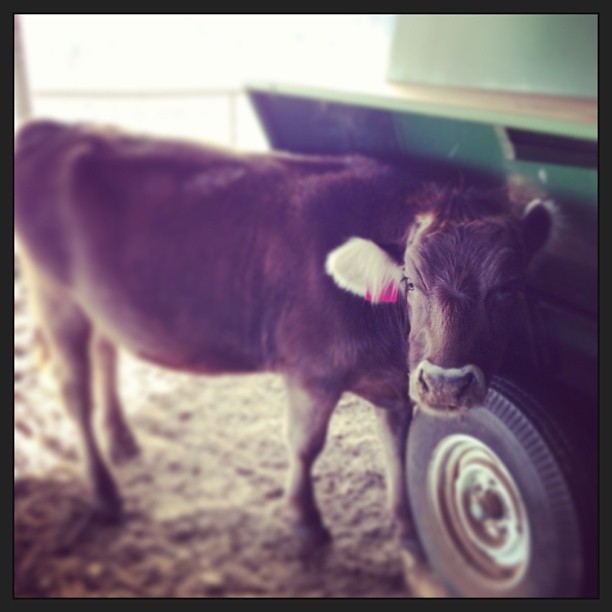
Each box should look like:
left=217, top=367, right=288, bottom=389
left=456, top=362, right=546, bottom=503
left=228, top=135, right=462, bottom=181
left=239, top=14, right=600, bottom=594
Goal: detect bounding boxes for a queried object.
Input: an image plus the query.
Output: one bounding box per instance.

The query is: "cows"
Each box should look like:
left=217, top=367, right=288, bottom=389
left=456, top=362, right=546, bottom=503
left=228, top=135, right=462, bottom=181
left=14, top=118, right=564, bottom=547
